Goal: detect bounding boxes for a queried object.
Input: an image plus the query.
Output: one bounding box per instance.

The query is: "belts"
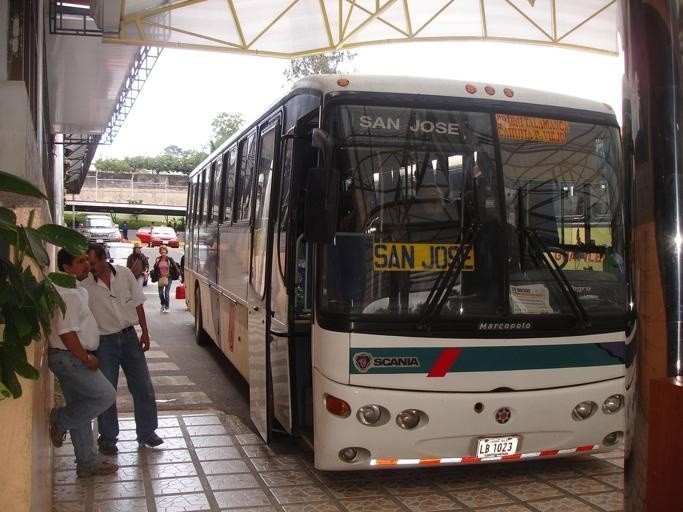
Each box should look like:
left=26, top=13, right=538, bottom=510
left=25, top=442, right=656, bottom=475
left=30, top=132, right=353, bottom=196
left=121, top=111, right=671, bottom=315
left=116, top=326, right=133, bottom=334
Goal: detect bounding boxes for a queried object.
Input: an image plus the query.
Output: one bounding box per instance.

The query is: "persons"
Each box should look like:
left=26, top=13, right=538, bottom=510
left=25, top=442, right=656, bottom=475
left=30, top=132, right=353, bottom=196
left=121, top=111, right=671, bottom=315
left=153, top=245, right=178, bottom=313
left=121, top=221, right=128, bottom=239
left=417, top=182, right=445, bottom=216
left=125, top=243, right=150, bottom=292
left=79, top=245, right=164, bottom=456
left=201, top=242, right=218, bottom=284
left=179, top=247, right=184, bottom=283
left=48, top=246, right=119, bottom=479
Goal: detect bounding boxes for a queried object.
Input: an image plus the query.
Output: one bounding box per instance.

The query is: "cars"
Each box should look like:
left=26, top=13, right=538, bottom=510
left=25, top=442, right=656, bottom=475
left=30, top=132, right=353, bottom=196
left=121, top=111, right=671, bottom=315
left=135, top=226, right=179, bottom=248
left=79, top=215, right=120, bottom=243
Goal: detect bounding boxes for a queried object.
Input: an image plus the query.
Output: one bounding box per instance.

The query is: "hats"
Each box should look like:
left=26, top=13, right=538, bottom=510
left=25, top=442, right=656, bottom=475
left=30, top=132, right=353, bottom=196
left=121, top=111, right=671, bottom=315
left=133, top=243, right=142, bottom=248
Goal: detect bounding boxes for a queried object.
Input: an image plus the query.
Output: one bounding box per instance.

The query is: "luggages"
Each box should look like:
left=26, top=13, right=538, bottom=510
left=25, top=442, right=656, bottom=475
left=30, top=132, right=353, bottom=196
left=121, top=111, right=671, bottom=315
left=176, top=282, right=185, bottom=299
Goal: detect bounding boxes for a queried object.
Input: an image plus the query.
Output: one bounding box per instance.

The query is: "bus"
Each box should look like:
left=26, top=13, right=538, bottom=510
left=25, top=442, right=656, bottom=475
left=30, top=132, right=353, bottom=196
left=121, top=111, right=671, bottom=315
left=182, top=72, right=629, bottom=481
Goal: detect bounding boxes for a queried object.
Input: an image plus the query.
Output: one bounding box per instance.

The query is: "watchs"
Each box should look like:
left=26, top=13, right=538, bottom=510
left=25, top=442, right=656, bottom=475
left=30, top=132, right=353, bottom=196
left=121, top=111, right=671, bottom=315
left=84, top=353, right=94, bottom=362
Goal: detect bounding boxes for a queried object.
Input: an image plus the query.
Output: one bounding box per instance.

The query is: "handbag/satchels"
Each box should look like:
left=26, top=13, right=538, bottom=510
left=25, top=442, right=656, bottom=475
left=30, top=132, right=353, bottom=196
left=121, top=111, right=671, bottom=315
left=159, top=277, right=169, bottom=287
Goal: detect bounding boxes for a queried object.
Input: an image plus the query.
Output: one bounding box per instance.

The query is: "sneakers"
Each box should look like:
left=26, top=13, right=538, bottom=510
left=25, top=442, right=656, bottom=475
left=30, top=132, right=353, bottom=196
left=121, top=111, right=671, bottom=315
left=138, top=431, right=163, bottom=447
left=99, top=445, right=118, bottom=455
left=77, top=460, right=118, bottom=477
left=160, top=304, right=169, bottom=313
left=49, top=407, right=63, bottom=448
